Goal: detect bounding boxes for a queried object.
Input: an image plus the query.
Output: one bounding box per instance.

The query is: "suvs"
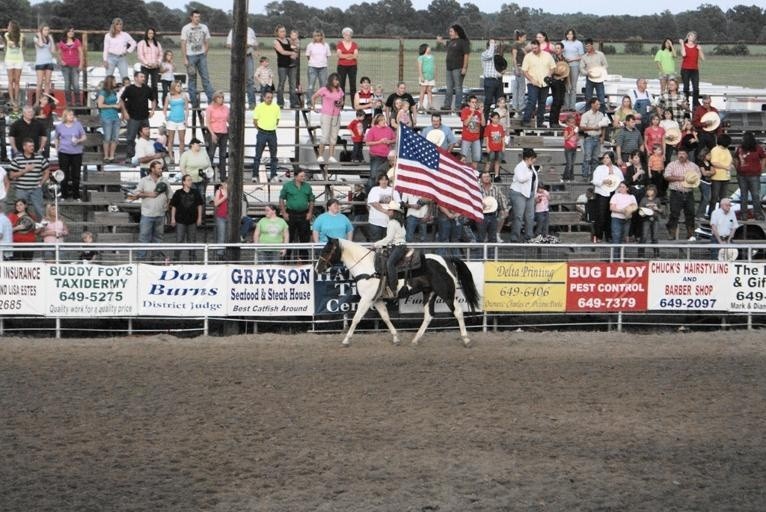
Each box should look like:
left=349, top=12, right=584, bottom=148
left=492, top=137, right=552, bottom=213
left=539, top=97, right=616, bottom=126
left=517, top=126, right=615, bottom=158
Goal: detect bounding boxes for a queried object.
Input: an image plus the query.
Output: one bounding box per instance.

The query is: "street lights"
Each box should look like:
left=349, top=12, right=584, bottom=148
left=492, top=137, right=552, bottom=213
left=46, top=164, right=69, bottom=340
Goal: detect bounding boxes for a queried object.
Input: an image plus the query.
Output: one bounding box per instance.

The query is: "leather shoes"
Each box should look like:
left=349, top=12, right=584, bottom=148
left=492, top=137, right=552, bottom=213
left=521, top=123, right=563, bottom=127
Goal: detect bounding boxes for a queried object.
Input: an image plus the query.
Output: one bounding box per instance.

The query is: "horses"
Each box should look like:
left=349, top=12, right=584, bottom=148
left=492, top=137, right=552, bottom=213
left=314, top=234, right=480, bottom=348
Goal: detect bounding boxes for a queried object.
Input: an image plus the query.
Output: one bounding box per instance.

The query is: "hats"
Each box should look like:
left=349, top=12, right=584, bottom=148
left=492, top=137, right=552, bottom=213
left=663, top=111, right=720, bottom=144
left=552, top=60, right=607, bottom=83
left=718, top=248, right=738, bottom=262
left=15, top=215, right=35, bottom=234
left=154, top=182, right=167, bottom=193
left=482, top=196, right=497, bottom=213
left=493, top=55, right=508, bottom=72
left=191, top=139, right=200, bottom=143
left=426, top=129, right=445, bottom=147
left=682, top=170, right=699, bottom=188
left=381, top=200, right=405, bottom=213
left=603, top=174, right=654, bottom=219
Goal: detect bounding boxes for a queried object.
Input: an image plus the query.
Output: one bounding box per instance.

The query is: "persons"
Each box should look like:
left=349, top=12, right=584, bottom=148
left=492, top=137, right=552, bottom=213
left=0, top=167, right=10, bottom=213
left=98, top=10, right=765, bottom=262
left=32, top=23, right=57, bottom=106
left=34, top=92, right=59, bottom=159
left=56, top=25, right=84, bottom=105
left=8, top=137, right=51, bottom=222
left=1, top=199, right=68, bottom=264
left=71, top=231, right=98, bottom=264
left=373, top=200, right=408, bottom=299
left=8, top=105, right=47, bottom=159
left=54, top=108, right=87, bottom=203
left=3, top=19, right=26, bottom=113
left=0, top=86, right=10, bottom=164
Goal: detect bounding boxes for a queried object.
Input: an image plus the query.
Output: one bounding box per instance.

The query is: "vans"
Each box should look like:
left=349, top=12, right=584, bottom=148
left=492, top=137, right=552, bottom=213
left=697, top=164, right=766, bottom=262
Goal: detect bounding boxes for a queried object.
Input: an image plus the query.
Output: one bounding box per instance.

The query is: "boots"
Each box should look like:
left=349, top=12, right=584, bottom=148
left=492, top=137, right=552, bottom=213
left=496, top=233, right=504, bottom=242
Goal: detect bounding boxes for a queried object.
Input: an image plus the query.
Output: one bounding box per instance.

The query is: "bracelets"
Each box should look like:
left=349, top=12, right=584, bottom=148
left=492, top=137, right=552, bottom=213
left=38, top=184, right=42, bottom=188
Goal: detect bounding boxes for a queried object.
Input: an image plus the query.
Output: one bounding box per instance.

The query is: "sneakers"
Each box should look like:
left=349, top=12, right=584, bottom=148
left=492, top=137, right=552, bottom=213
left=270, top=176, right=282, bottom=182
left=316, top=156, right=324, bottom=161
left=328, top=155, right=337, bottom=161
left=493, top=176, right=501, bottom=182
left=251, top=176, right=257, bottom=182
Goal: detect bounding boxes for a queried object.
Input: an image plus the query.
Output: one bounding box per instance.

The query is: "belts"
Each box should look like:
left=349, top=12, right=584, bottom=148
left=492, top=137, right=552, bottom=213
left=670, top=189, right=692, bottom=194
left=483, top=213, right=494, bottom=215
